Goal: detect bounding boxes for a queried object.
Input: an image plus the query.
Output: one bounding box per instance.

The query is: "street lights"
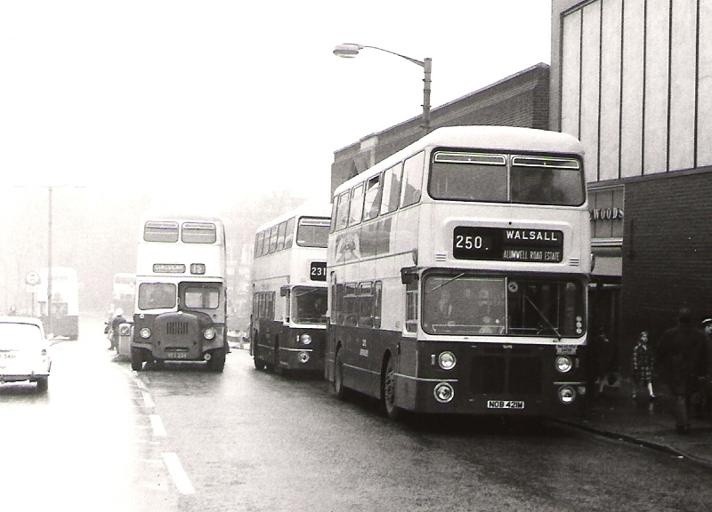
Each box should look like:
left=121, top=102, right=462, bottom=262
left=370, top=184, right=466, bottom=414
left=47, top=184, right=83, bottom=340
left=332, top=42, right=432, bottom=137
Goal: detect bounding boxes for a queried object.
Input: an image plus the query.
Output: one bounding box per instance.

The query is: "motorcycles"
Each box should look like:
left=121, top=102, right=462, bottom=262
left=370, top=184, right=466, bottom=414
left=105, top=320, right=121, bottom=353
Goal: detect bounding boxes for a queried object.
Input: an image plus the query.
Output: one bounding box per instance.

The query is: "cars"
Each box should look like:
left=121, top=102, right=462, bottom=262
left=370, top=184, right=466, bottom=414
left=0, top=315, right=51, bottom=393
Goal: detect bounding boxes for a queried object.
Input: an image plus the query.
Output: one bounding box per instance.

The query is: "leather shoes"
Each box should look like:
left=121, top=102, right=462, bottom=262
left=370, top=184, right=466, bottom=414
left=649, top=395, right=656, bottom=399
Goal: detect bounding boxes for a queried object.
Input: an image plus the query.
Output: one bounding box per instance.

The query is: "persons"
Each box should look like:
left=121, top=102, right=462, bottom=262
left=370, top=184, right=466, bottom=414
left=631, top=331, right=656, bottom=401
left=525, top=170, right=566, bottom=202
left=430, top=289, right=461, bottom=320
left=299, top=293, right=323, bottom=318
left=108, top=308, right=126, bottom=353
left=655, top=308, right=711, bottom=435
left=593, top=329, right=619, bottom=393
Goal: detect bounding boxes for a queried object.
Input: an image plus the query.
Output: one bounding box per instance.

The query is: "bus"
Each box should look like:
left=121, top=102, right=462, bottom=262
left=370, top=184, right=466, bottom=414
left=35, top=266, right=79, bottom=342
left=248, top=208, right=331, bottom=377
left=323, top=125, right=598, bottom=425
left=130, top=218, right=228, bottom=371
left=112, top=272, right=134, bottom=314
